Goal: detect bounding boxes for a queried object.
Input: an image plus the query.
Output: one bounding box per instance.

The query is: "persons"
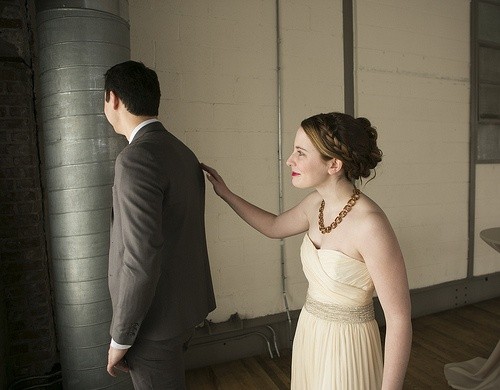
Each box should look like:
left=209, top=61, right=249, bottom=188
left=200, top=112, right=412, bottom=390
left=103, top=60, right=217, bottom=390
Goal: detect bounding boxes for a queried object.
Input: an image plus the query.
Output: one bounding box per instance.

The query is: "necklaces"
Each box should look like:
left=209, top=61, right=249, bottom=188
left=318, top=187, right=360, bottom=234
